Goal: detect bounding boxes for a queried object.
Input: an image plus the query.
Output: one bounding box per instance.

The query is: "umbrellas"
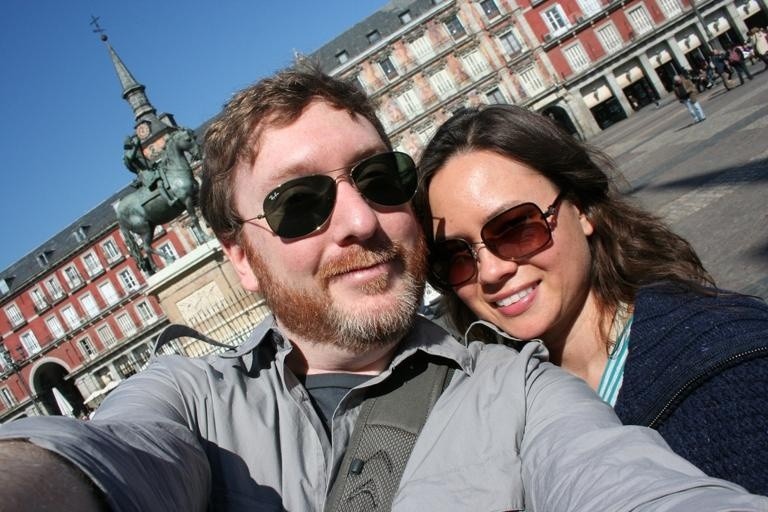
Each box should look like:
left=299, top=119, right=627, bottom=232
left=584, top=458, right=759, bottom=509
left=102, top=379, right=125, bottom=395
left=83, top=390, right=103, bottom=406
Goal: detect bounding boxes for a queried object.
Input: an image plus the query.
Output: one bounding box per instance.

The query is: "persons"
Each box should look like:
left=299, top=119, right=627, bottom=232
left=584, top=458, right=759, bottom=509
left=121, top=132, right=178, bottom=207
left=87, top=407, right=95, bottom=421
left=78, top=412, right=87, bottom=421
left=415, top=104, right=767, bottom=495
left=0, top=54, right=767, bottom=512
left=671, top=25, right=768, bottom=124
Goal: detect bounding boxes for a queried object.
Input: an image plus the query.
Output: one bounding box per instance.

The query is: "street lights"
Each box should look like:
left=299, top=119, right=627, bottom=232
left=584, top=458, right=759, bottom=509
left=0, top=334, right=46, bottom=416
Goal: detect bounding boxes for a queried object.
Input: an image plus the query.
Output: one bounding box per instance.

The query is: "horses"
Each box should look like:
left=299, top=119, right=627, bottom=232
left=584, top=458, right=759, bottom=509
left=114, top=124, right=206, bottom=276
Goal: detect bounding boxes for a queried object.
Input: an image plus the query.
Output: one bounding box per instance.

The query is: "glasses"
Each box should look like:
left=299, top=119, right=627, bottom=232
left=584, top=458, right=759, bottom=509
left=238, top=150, right=421, bottom=240
left=426, top=189, right=565, bottom=288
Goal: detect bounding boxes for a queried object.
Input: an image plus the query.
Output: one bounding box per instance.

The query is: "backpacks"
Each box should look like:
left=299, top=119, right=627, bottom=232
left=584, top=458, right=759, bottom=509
left=727, top=48, right=741, bottom=62
